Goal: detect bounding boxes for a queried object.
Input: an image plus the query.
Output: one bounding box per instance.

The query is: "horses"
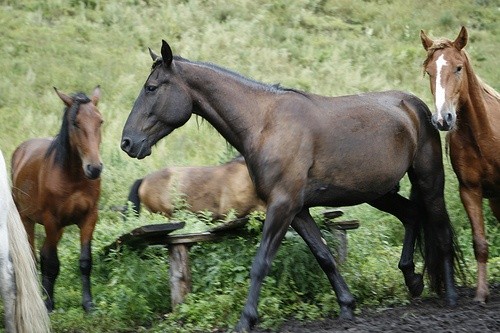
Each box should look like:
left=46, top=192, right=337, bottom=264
left=124, top=153, right=268, bottom=231
left=120, top=38, right=477, bottom=333
left=419, top=24, right=500, bottom=309
left=10, top=86, right=106, bottom=316
